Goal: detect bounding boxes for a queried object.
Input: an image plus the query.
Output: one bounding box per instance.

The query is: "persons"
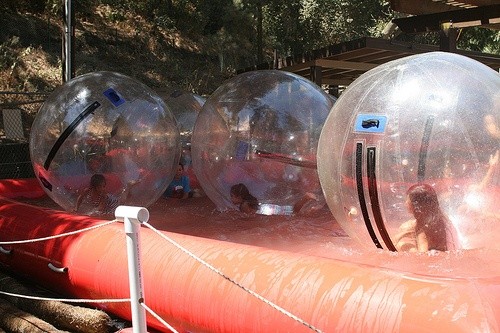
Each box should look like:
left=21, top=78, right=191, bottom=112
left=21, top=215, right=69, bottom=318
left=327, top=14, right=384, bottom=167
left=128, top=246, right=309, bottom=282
left=157, top=163, right=206, bottom=200
left=443, top=149, right=500, bottom=220
left=71, top=174, right=119, bottom=216
left=227, top=184, right=316, bottom=215
left=393, top=183, right=463, bottom=255
